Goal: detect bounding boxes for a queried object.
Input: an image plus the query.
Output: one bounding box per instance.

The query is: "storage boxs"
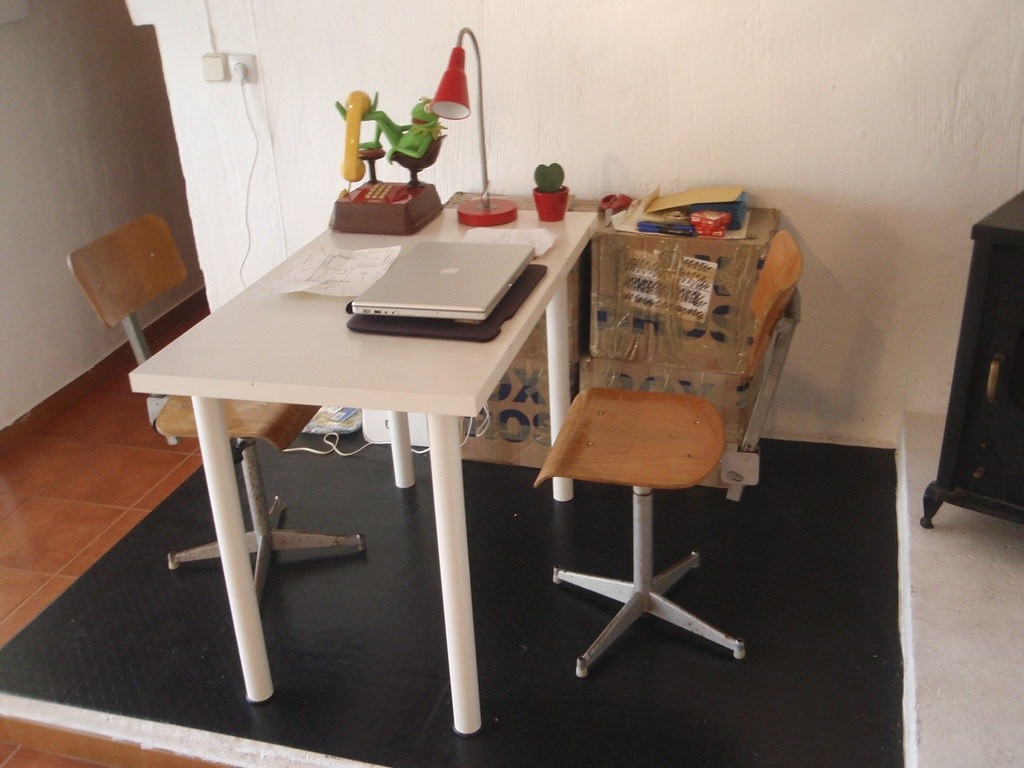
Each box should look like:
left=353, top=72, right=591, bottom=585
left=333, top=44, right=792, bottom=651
left=445, top=189, right=780, bottom=490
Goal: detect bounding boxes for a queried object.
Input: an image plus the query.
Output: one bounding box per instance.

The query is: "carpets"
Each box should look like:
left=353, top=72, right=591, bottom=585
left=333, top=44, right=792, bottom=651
left=0, top=426, right=906, bottom=768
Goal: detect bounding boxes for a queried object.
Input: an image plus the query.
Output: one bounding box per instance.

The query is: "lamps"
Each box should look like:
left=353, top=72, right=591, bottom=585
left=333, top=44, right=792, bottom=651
left=429, top=27, right=518, bottom=227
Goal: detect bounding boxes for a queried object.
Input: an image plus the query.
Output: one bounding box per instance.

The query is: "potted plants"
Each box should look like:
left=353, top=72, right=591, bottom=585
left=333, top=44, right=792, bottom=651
left=534, top=162, right=571, bottom=220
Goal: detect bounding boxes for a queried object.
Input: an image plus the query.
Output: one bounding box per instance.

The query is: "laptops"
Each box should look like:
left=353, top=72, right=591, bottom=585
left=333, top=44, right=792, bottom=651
left=352, top=242, right=536, bottom=320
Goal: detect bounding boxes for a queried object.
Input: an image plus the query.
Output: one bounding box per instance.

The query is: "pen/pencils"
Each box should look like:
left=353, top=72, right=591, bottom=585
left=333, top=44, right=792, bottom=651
left=634, top=221, right=697, bottom=237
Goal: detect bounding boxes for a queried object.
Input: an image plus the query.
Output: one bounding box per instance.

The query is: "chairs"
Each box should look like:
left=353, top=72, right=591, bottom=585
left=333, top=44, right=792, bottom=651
left=533, top=230, right=804, bottom=678
left=68, top=215, right=368, bottom=603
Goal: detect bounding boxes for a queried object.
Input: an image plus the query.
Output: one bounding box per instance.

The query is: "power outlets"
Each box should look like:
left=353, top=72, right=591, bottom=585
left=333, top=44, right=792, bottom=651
left=227, top=54, right=257, bottom=84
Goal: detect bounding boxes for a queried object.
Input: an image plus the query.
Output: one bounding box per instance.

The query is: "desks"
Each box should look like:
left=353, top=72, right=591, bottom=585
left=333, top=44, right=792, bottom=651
left=129, top=204, right=599, bottom=736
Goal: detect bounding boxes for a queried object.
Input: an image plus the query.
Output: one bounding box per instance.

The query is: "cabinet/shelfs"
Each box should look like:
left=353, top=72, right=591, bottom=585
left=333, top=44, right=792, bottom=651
left=920, top=191, right=1023, bottom=527
